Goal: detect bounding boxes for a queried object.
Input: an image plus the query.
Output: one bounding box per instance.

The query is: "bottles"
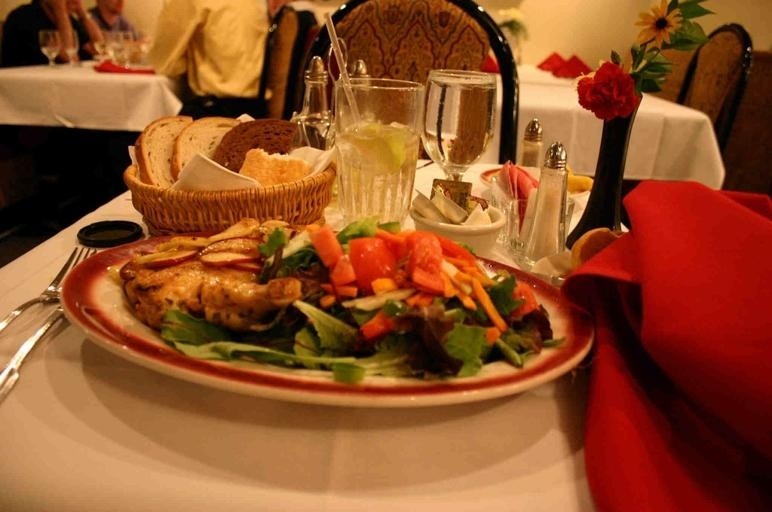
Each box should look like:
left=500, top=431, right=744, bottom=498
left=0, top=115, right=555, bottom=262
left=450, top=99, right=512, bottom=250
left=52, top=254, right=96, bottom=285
left=523, top=143, right=568, bottom=267
left=299, top=57, right=332, bottom=136
left=520, top=119, right=542, bottom=166
left=348, top=59, right=373, bottom=118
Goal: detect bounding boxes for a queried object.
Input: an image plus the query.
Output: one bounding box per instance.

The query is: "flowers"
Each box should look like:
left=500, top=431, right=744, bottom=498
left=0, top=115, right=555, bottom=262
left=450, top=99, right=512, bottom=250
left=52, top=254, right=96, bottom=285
left=576, top=0, right=719, bottom=122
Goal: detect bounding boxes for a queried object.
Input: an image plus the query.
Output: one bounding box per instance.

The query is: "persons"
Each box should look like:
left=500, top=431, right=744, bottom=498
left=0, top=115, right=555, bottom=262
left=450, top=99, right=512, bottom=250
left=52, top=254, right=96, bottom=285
left=149, top=0, right=270, bottom=119
left=0, top=0, right=102, bottom=230
left=79, top=0, right=146, bottom=45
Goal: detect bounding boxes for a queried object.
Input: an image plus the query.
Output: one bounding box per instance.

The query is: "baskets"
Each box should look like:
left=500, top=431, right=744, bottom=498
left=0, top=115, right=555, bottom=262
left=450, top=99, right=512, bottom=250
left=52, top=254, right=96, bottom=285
left=123, top=161, right=338, bottom=238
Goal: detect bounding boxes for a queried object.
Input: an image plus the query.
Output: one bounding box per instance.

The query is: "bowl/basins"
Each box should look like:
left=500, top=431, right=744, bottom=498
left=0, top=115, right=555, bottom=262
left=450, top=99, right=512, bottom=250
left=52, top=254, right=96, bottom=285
left=409, top=202, right=505, bottom=257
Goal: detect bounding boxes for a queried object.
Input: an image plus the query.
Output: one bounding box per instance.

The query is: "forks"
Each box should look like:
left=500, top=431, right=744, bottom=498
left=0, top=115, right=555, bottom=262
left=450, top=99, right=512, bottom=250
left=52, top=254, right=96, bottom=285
left=0, top=246, right=98, bottom=332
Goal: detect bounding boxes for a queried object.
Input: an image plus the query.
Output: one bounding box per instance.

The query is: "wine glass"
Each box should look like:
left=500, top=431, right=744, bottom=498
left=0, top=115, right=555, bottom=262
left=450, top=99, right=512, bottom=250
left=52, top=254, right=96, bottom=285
left=40, top=30, right=153, bottom=69
left=421, top=70, right=497, bottom=182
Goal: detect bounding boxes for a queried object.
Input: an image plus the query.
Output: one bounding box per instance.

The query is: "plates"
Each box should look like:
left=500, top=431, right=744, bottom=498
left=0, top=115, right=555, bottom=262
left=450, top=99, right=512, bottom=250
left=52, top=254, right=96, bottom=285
left=61, top=231, right=596, bottom=410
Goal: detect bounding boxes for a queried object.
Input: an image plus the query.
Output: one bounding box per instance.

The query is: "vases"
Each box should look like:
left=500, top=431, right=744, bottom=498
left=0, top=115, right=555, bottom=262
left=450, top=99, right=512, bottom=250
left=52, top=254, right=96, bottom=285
left=563, top=91, right=643, bottom=253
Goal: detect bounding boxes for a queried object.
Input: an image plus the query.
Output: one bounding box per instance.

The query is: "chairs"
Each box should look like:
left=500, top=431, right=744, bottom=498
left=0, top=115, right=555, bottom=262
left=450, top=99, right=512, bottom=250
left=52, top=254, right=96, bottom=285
left=300, top=3, right=522, bottom=177
left=247, top=6, right=302, bottom=123
left=674, top=22, right=753, bottom=150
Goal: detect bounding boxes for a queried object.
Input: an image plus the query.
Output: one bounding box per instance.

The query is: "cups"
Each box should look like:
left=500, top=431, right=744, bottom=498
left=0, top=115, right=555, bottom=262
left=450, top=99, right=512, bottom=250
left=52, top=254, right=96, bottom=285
left=333, top=79, right=426, bottom=229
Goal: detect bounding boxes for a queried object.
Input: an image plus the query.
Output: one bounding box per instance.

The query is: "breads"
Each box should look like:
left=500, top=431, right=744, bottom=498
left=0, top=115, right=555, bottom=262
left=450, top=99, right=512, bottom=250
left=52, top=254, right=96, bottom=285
left=135, top=115, right=304, bottom=189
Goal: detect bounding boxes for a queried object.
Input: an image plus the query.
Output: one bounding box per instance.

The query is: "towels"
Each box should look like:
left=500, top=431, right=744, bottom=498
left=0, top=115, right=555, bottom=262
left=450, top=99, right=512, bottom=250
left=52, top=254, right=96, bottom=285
left=92, top=58, right=154, bottom=76
left=536, top=50, right=566, bottom=72
left=552, top=52, right=593, bottom=80
left=478, top=53, right=501, bottom=72
left=560, top=177, right=771, bottom=512
left=496, top=160, right=538, bottom=230
